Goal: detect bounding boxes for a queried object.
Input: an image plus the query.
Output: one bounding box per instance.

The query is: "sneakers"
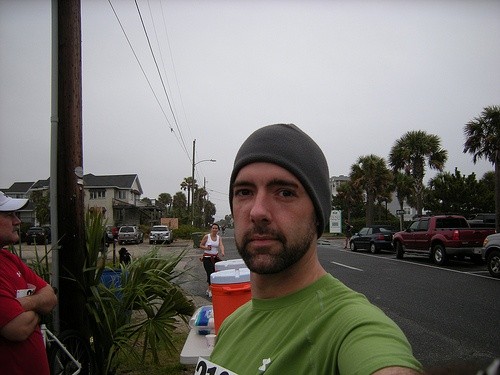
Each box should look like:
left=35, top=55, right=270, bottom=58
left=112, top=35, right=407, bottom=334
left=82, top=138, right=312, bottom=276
left=206, top=289, right=212, bottom=297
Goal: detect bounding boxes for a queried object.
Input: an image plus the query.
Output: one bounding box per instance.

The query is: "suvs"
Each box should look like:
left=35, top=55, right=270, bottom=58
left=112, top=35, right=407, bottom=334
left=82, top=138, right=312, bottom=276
left=25, top=227, right=47, bottom=245
left=105, top=225, right=119, bottom=240
left=118, top=226, right=144, bottom=245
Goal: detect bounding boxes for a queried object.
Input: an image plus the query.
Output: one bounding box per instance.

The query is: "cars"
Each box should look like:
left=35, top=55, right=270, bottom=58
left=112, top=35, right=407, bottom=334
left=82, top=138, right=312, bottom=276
left=149, top=225, right=174, bottom=244
left=349, top=227, right=396, bottom=254
left=44, top=226, right=51, bottom=242
left=481, top=233, right=500, bottom=278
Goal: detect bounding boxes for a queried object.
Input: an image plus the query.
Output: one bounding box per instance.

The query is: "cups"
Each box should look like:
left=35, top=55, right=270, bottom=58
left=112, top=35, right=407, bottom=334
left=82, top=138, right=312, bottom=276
left=205, top=335, right=216, bottom=349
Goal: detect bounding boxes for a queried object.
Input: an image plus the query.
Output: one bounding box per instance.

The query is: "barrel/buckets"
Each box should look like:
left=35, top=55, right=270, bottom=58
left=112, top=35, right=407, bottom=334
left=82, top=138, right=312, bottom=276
left=214, top=259, right=247, bottom=272
left=99, top=270, right=133, bottom=318
left=210, top=268, right=251, bottom=336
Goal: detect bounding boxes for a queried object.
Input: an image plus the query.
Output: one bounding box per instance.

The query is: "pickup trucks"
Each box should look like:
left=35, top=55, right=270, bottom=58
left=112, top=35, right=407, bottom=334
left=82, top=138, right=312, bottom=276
left=392, top=215, right=497, bottom=266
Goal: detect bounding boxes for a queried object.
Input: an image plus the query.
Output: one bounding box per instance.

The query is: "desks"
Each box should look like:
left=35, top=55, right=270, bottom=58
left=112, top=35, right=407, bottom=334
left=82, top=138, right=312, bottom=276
left=179, top=305, right=216, bottom=364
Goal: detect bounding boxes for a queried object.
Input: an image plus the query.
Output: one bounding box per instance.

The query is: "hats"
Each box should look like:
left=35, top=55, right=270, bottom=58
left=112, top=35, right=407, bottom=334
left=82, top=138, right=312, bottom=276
left=229, top=122, right=331, bottom=238
left=0, top=190, right=30, bottom=211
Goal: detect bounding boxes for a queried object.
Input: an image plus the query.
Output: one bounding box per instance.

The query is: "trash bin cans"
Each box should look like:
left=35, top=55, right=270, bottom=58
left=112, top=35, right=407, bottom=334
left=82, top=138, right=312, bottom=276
left=100, top=269, right=131, bottom=299
left=210, top=259, right=251, bottom=335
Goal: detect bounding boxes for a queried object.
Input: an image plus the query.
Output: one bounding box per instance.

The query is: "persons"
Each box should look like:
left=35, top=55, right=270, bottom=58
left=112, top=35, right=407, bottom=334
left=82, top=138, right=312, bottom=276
left=194, top=123, right=424, bottom=375
left=0, top=191, right=57, bottom=375
left=221, top=224, right=226, bottom=234
left=102, top=227, right=113, bottom=253
left=199, top=223, right=225, bottom=298
left=344, top=220, right=353, bottom=249
left=118, top=246, right=131, bottom=270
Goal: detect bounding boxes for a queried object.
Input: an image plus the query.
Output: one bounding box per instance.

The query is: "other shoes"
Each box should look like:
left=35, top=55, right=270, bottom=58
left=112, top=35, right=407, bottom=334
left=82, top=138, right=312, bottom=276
left=343, top=244, right=348, bottom=250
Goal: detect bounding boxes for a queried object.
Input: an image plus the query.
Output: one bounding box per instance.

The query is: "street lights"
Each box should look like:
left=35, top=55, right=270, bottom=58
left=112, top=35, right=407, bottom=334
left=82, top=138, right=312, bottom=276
left=191, top=140, right=216, bottom=226
